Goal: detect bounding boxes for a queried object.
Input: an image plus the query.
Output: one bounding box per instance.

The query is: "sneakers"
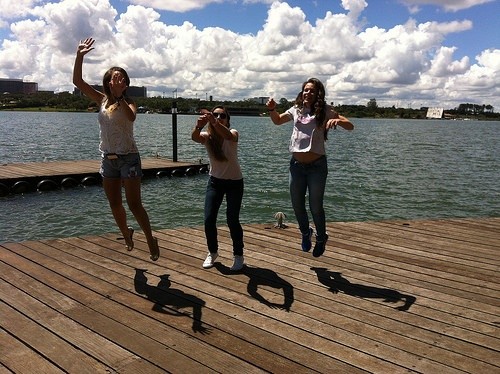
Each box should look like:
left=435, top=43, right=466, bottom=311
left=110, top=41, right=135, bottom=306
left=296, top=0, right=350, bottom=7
left=313, top=231, right=329, bottom=257
left=230, top=255, right=244, bottom=271
left=202, top=251, right=218, bottom=268
left=301, top=226, right=313, bottom=252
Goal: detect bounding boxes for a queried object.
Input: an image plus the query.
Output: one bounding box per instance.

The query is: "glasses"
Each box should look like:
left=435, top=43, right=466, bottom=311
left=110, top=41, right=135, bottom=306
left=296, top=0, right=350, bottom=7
left=212, top=112, right=228, bottom=119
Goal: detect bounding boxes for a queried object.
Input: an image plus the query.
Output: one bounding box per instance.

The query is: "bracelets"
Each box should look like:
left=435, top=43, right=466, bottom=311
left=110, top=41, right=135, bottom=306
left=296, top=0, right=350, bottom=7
left=114, top=95, right=123, bottom=103
left=269, top=109, right=274, bottom=111
left=196, top=126, right=202, bottom=130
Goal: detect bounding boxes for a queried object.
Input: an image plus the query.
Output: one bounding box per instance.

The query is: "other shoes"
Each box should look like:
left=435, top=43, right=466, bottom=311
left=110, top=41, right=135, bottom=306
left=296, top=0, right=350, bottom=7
left=124, top=227, right=134, bottom=251
left=149, top=236, right=160, bottom=261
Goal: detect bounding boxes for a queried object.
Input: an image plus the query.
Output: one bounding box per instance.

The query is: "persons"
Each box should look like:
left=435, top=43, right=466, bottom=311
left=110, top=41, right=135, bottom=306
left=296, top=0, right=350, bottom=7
left=191, top=105, right=244, bottom=271
left=73, top=37, right=160, bottom=261
left=266, top=78, right=354, bottom=257
